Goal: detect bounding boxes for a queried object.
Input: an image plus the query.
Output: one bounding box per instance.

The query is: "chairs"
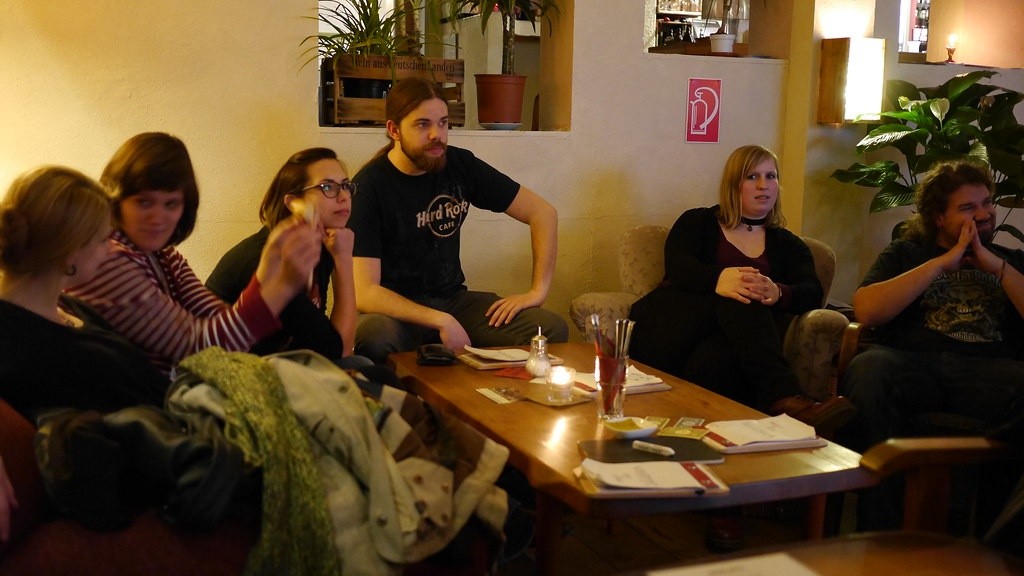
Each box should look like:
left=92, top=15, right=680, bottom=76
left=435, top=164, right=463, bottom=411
left=837, top=320, right=1023, bottom=532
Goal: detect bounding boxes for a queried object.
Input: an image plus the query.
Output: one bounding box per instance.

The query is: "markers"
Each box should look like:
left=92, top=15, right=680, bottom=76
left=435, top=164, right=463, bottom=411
left=632, top=440, right=675, bottom=456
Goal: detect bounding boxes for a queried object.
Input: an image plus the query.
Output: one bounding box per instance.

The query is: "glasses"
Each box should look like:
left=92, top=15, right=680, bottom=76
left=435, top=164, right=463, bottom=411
left=303, top=181, right=358, bottom=198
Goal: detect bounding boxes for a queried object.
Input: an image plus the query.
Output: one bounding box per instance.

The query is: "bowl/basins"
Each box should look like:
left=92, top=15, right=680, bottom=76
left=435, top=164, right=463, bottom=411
left=604, top=417, right=658, bottom=439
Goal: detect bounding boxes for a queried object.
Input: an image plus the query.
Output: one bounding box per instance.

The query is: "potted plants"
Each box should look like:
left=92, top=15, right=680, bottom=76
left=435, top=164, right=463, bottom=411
left=449, top=0, right=555, bottom=129
left=298, top=0, right=466, bottom=128
left=705, top=0, right=767, bottom=53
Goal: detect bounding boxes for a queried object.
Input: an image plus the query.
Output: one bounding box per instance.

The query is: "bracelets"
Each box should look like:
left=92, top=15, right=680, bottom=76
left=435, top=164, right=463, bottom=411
left=999, top=258, right=1006, bottom=282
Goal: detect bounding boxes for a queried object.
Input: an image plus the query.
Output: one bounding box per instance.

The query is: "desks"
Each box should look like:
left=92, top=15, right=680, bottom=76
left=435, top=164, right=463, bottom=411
left=390, top=345, right=878, bottom=576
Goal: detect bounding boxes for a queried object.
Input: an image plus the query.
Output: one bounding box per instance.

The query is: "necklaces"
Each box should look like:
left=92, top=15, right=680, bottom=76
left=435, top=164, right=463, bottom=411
left=737, top=216, right=767, bottom=232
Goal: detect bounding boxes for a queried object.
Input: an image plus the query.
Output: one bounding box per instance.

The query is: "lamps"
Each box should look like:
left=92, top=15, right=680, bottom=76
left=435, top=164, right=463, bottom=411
left=945, top=36, right=957, bottom=64
left=547, top=367, right=577, bottom=408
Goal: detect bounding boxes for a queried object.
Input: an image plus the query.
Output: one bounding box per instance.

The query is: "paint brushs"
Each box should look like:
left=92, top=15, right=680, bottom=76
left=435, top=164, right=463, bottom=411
left=590, top=314, right=636, bottom=357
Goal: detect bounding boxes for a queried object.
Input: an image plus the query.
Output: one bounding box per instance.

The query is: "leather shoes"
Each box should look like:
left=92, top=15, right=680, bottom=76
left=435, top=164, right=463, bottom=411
left=705, top=508, right=744, bottom=549
left=771, top=392, right=858, bottom=431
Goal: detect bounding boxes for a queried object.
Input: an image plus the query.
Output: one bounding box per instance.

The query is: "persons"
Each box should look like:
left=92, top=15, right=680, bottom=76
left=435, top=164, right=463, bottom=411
left=62, top=132, right=321, bottom=363
left=345, top=76, right=568, bottom=354
left=204, top=148, right=377, bottom=375
left=0, top=164, right=175, bottom=429
left=627, top=145, right=822, bottom=552
left=838, top=164, right=1024, bottom=558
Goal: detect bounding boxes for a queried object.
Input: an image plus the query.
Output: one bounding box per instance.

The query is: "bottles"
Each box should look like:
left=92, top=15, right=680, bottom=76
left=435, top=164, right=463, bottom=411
left=658, top=0, right=699, bottom=12
left=683, top=28, right=692, bottom=43
left=913, top=0, right=930, bottom=40
left=526, top=335, right=551, bottom=377
left=677, top=28, right=683, bottom=41
left=919, top=41, right=927, bottom=52
left=700, top=29, right=704, bottom=39
left=669, top=29, right=674, bottom=44
left=659, top=32, right=664, bottom=46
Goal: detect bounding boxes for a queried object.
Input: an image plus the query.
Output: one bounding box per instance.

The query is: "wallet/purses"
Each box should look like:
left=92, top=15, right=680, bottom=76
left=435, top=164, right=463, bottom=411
left=416, top=343, right=457, bottom=365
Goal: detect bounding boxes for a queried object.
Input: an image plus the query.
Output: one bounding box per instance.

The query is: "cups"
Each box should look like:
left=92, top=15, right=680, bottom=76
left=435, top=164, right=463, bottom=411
left=596, top=355, right=629, bottom=421
left=908, top=41, right=919, bottom=53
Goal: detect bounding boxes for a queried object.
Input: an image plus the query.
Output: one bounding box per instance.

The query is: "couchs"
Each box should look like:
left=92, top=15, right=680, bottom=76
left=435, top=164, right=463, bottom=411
left=0, top=398, right=259, bottom=576
left=566, top=225, right=850, bottom=407
